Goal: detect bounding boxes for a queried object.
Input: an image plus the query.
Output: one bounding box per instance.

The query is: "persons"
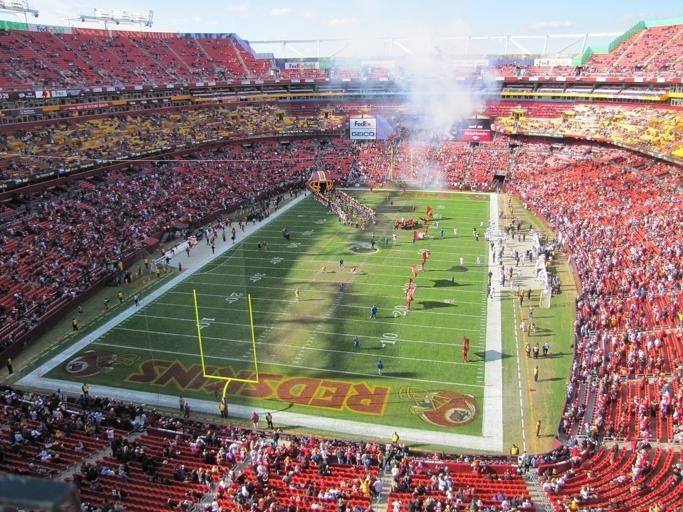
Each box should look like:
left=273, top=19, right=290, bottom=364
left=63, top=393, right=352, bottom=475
left=1, top=23, right=682, bottom=512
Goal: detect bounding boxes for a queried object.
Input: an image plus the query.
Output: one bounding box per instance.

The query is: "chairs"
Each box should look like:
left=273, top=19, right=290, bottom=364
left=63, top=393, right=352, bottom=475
left=500, top=20, right=681, bottom=375
left=0, top=376, right=682, bottom=512
left=278, top=60, right=510, bottom=195
left=0, top=20, right=306, bottom=360
